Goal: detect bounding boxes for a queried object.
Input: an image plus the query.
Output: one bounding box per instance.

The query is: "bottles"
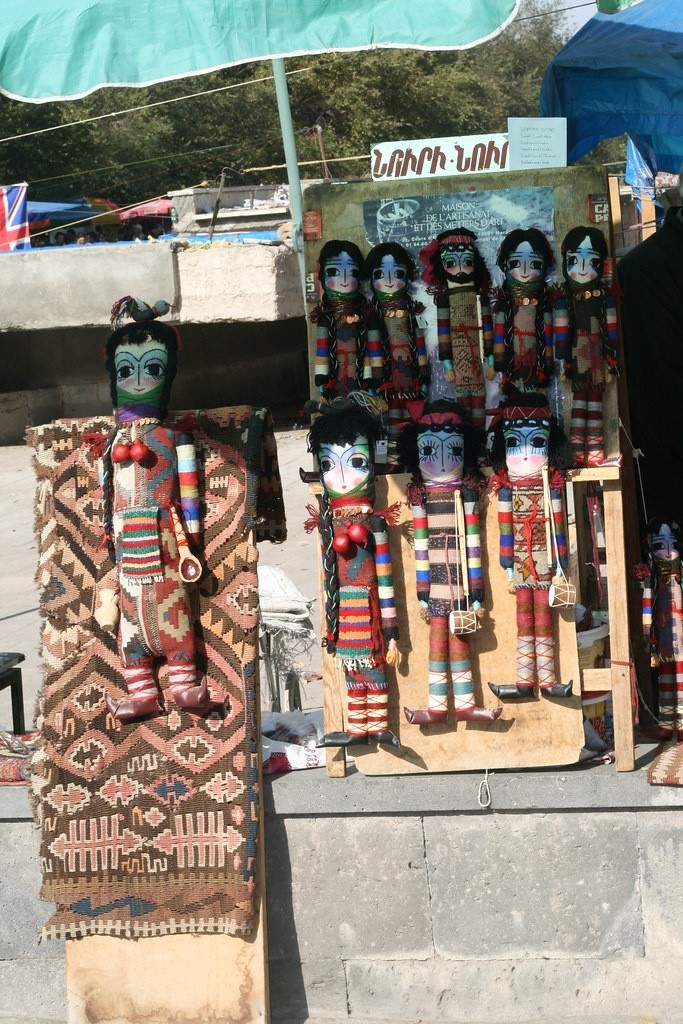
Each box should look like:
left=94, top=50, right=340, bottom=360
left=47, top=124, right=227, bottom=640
left=170, top=206, right=178, bottom=223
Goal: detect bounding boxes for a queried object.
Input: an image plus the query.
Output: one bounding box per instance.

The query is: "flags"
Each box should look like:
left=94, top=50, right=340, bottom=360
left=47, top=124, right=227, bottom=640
left=0, top=182, right=31, bottom=252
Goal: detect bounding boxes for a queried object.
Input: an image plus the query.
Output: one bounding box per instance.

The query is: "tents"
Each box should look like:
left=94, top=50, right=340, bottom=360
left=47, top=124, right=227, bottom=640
left=26, top=198, right=123, bottom=233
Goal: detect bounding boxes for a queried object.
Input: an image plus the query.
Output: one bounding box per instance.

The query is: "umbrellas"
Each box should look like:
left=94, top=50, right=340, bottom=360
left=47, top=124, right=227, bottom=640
left=119, top=198, right=172, bottom=221
left=1, top=0, right=524, bottom=308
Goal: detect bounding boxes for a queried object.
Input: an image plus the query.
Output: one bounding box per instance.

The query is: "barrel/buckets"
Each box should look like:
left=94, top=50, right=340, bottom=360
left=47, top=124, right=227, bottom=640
left=576, top=624, right=609, bottom=742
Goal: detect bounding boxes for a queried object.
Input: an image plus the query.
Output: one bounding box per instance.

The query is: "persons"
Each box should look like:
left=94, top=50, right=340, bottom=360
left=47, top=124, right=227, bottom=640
left=614, top=200, right=682, bottom=560
left=26, top=223, right=166, bottom=247
left=92, top=223, right=683, bottom=749
